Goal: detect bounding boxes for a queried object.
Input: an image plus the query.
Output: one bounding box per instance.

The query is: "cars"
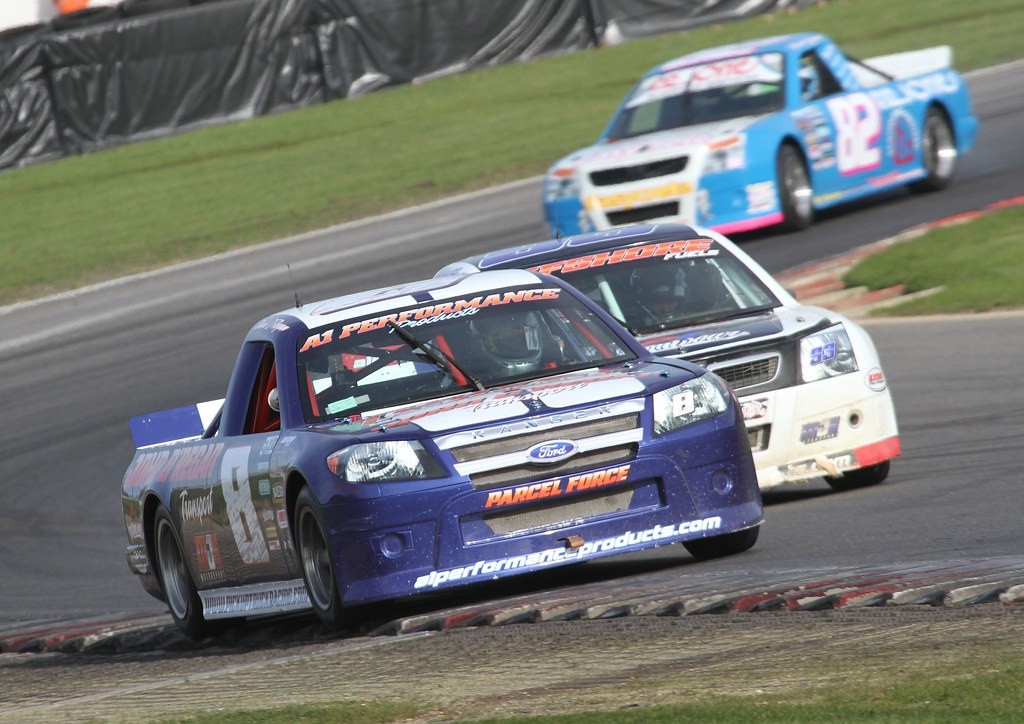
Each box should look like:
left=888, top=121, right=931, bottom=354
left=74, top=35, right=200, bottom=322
left=119, top=268, right=768, bottom=635
left=541, top=31, right=978, bottom=237
left=432, top=222, right=902, bottom=490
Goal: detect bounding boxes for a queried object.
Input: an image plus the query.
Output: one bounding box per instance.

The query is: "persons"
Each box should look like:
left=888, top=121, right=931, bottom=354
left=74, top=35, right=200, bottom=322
left=626, top=263, right=689, bottom=325
left=469, top=303, right=547, bottom=378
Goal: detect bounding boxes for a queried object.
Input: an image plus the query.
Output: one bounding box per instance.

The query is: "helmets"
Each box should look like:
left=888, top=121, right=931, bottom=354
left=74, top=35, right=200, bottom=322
left=470, top=310, right=542, bottom=378
left=800, top=64, right=820, bottom=99
left=630, top=265, right=688, bottom=325
